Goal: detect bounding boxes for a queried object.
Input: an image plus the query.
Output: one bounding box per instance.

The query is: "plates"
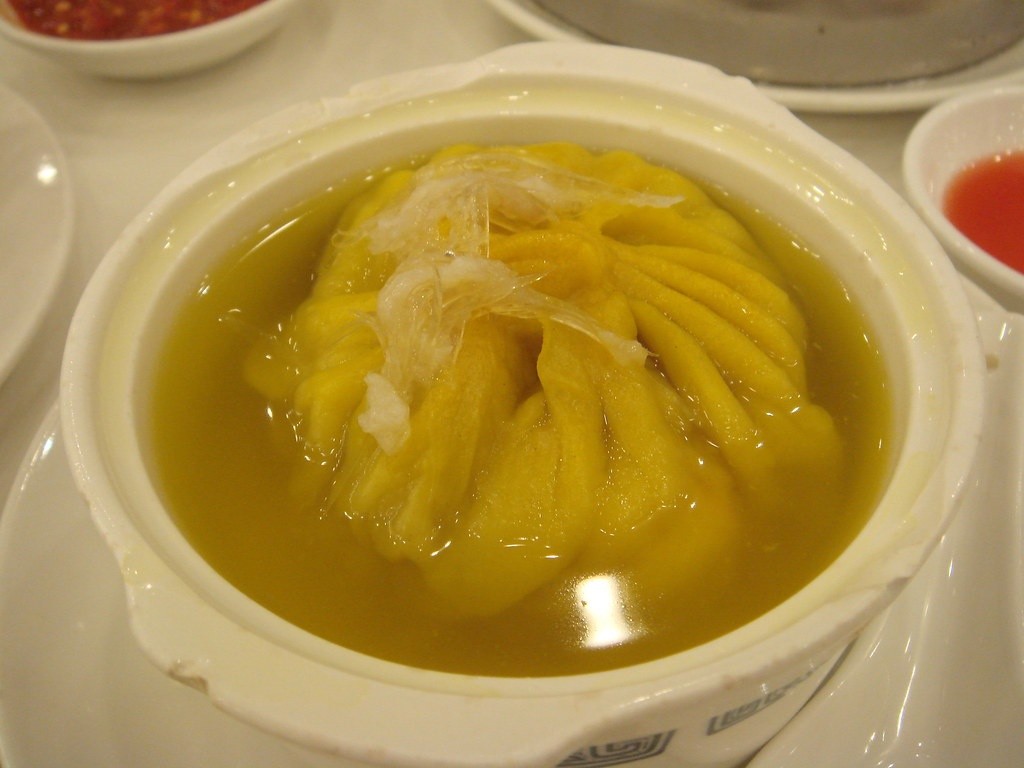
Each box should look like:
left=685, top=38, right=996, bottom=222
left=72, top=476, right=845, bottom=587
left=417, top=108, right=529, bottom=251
left=0, top=88, right=77, bottom=386
left=488, top=0, right=1024, bottom=115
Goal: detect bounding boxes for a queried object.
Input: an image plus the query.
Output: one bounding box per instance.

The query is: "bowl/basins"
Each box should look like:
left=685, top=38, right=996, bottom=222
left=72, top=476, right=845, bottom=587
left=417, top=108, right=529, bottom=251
left=0, top=0, right=294, bottom=81
left=65, top=43, right=983, bottom=768
left=903, top=84, right=1024, bottom=298
